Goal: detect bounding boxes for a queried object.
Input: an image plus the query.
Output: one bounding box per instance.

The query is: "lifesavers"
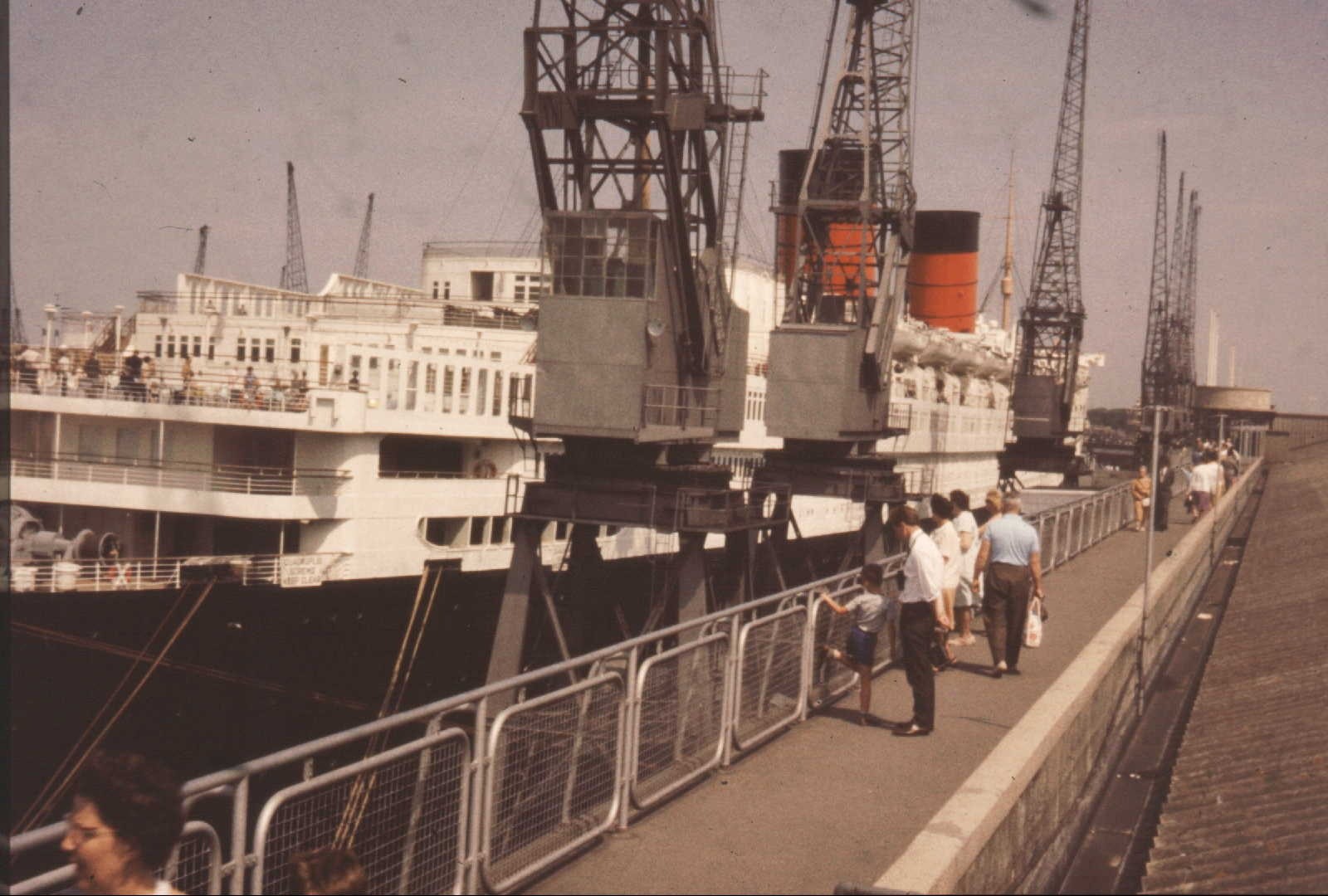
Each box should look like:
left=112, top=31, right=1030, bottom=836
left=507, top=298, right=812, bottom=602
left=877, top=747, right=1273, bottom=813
left=106, top=562, right=134, bottom=587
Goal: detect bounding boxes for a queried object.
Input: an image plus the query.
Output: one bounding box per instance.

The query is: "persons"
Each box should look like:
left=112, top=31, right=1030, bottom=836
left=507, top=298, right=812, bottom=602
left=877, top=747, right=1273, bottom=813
left=972, top=493, right=1044, bottom=678
left=1154, top=456, right=1174, bottom=531
left=244, top=367, right=257, bottom=400
left=126, top=351, right=160, bottom=387
left=41, top=751, right=192, bottom=896
left=1132, top=466, right=1151, bottom=531
left=978, top=491, right=1002, bottom=534
left=889, top=505, right=950, bottom=736
left=931, top=493, right=961, bottom=665
left=289, top=850, right=367, bottom=896
left=12, top=345, right=41, bottom=394
left=84, top=353, right=99, bottom=378
left=1182, top=438, right=1239, bottom=524
left=349, top=371, right=358, bottom=389
left=822, top=563, right=898, bottom=726
left=292, top=370, right=308, bottom=391
left=950, top=489, right=979, bottom=645
left=183, top=357, right=193, bottom=391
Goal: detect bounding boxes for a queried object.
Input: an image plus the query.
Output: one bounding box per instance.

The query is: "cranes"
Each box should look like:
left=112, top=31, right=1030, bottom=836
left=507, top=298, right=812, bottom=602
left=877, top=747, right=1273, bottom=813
left=758, top=1, right=931, bottom=545
left=1139, top=121, right=1208, bottom=480
left=513, top=0, right=764, bottom=537
left=265, top=160, right=307, bottom=291
left=996, top=0, right=1113, bottom=490
left=352, top=189, right=373, bottom=279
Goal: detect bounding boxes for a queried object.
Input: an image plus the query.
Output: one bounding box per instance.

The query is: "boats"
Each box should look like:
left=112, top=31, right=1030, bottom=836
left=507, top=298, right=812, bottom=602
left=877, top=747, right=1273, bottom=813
left=1, top=235, right=1104, bottom=896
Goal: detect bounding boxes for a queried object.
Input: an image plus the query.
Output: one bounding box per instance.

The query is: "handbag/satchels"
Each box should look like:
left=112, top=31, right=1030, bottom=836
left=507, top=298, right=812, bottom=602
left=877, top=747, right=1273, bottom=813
left=1021, top=595, right=1044, bottom=649
left=1142, top=497, right=1151, bottom=508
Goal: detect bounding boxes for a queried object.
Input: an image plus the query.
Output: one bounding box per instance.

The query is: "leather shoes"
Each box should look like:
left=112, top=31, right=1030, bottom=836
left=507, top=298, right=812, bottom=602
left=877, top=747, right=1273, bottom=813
left=893, top=717, right=934, bottom=737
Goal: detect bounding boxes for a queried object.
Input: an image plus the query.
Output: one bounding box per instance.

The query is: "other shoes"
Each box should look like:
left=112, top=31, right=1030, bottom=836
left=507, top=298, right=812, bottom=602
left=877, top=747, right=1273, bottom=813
left=1006, top=668, right=1022, bottom=676
left=948, top=634, right=977, bottom=647
left=1135, top=521, right=1146, bottom=531
left=994, top=659, right=1009, bottom=679
left=1191, top=513, right=1199, bottom=524
left=817, top=644, right=834, bottom=667
left=932, top=655, right=958, bottom=673
left=860, top=713, right=877, bottom=726
